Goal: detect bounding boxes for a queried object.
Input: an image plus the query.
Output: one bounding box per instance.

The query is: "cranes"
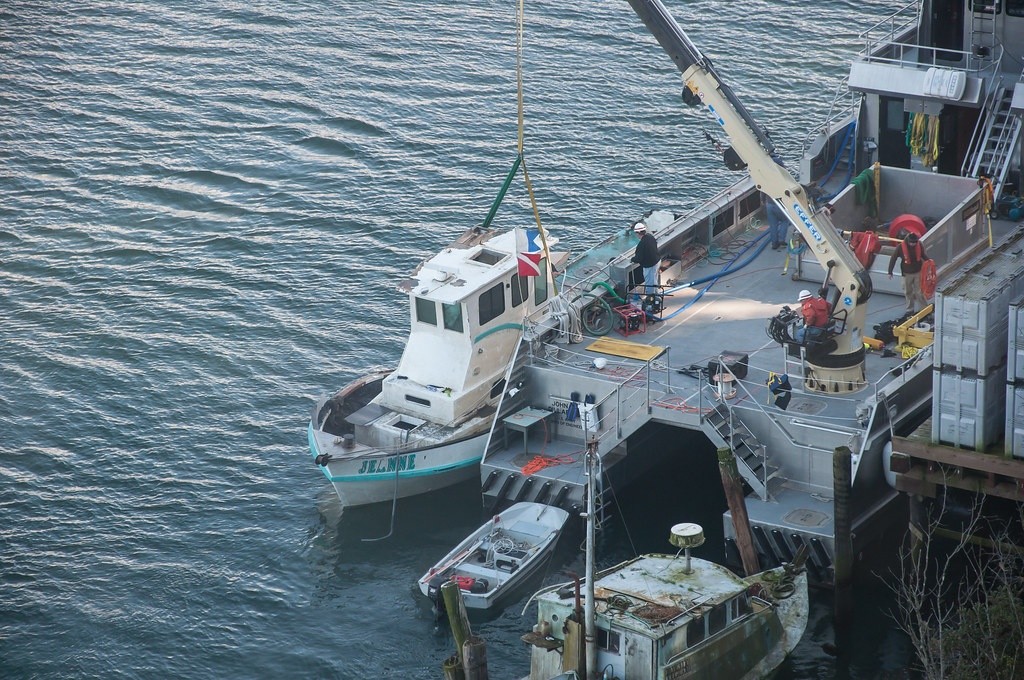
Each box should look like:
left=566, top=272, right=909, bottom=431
left=483, top=0, right=873, bottom=395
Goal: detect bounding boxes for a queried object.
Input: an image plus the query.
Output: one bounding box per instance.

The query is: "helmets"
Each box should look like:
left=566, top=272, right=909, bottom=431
left=633, top=223, right=647, bottom=232
left=906, top=232, right=918, bottom=243
left=796, top=289, right=812, bottom=301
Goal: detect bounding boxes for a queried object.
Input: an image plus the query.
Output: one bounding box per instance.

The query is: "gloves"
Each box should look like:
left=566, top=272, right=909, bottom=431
left=803, top=324, right=807, bottom=329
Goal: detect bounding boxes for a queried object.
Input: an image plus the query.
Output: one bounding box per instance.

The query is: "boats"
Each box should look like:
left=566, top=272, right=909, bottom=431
left=481, top=0, right=1024, bottom=594
left=517, top=435, right=809, bottom=680
left=308, top=211, right=688, bottom=506
left=418, top=502, right=570, bottom=609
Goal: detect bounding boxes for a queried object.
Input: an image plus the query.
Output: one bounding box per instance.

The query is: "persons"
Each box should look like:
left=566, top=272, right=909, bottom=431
left=630, top=223, right=662, bottom=298
left=797, top=290, right=832, bottom=341
left=887, top=232, right=934, bottom=315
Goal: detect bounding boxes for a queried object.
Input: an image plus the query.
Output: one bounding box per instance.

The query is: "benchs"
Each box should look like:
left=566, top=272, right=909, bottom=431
left=480, top=542, right=528, bottom=565
left=450, top=562, right=510, bottom=591
left=494, top=520, right=550, bottom=546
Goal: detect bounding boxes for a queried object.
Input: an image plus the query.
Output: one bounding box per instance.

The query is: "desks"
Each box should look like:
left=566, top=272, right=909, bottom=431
left=502, top=406, right=554, bottom=455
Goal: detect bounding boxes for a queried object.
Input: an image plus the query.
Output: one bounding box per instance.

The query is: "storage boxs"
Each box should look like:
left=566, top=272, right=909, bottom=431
left=707, top=350, right=748, bottom=387
left=656, top=258, right=682, bottom=286
